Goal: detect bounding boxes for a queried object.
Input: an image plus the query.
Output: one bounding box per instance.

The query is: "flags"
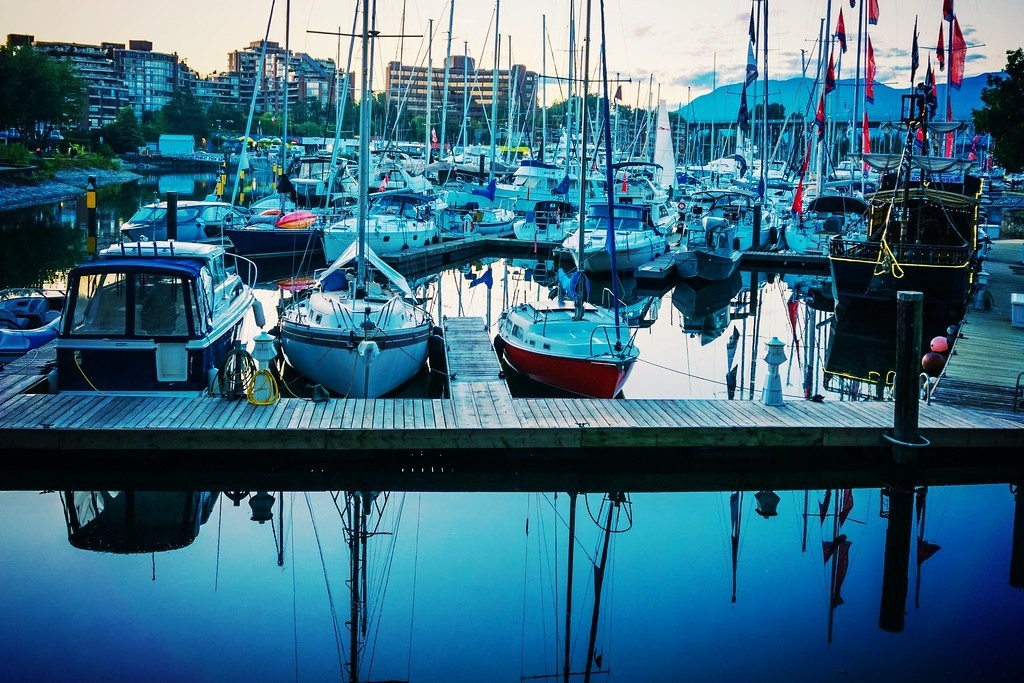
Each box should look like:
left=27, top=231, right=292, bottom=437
left=724, top=326, right=743, bottom=400
left=621, top=174, right=628, bottom=192
left=735, top=83, right=749, bottom=134
left=734, top=122, right=747, bottom=178
left=747, top=10, right=760, bottom=44
left=791, top=139, right=812, bottom=215
left=744, top=38, right=758, bottom=87
left=824, top=52, right=836, bottom=95
left=834, top=7, right=848, bottom=54
left=787, top=291, right=800, bottom=331
left=801, top=372, right=810, bottom=400
left=866, top=35, right=876, bottom=105
left=910, top=0, right=995, bottom=172
left=869, top=0, right=880, bottom=25
left=814, top=92, right=827, bottom=142
left=862, top=109, right=872, bottom=178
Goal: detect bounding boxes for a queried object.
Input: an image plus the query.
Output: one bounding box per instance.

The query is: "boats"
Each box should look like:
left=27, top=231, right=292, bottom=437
left=118, top=1, right=1022, bottom=323
left=0, top=284, right=67, bottom=355
left=277, top=0, right=438, bottom=399
left=491, top=0, right=640, bottom=399
left=52, top=192, right=260, bottom=398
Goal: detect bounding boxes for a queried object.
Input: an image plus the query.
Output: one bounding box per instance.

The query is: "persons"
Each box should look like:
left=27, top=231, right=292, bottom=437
left=666, top=185, right=674, bottom=201
left=276, top=174, right=296, bottom=203
